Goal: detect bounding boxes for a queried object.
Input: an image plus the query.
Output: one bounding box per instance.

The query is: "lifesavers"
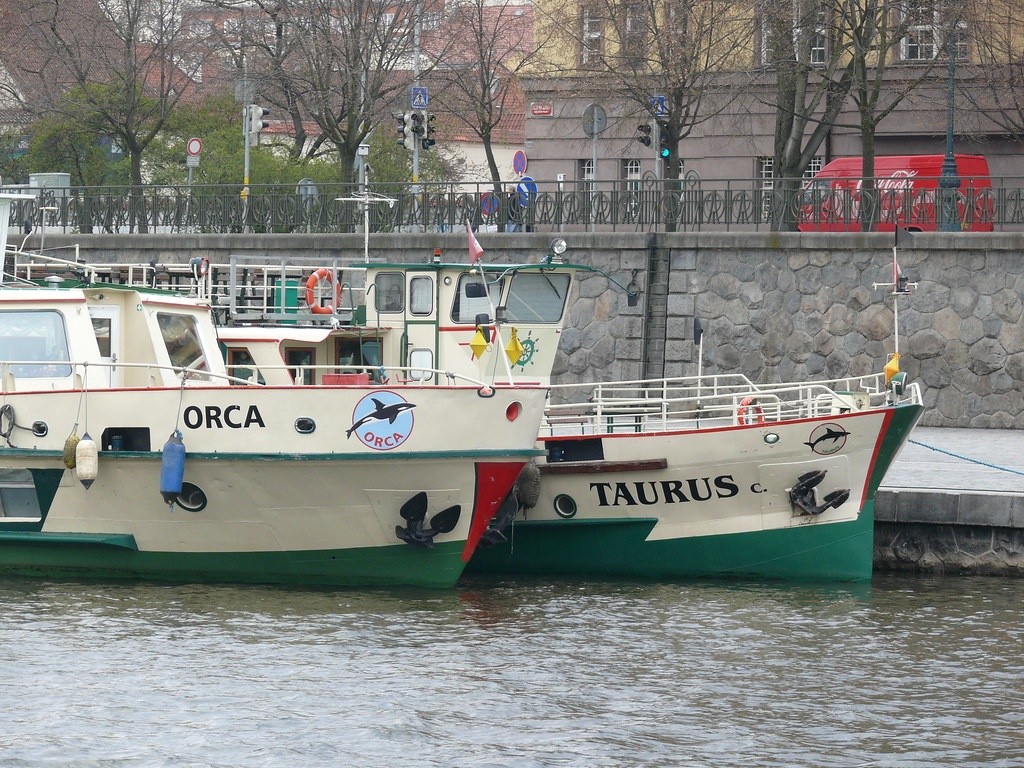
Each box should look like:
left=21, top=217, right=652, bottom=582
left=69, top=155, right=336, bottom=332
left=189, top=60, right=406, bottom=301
left=737, top=395, right=764, bottom=425
left=305, top=268, right=343, bottom=315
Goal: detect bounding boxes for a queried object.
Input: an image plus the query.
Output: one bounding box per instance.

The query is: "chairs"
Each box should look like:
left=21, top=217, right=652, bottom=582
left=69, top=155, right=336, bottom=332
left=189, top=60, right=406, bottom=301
left=386, top=283, right=403, bottom=311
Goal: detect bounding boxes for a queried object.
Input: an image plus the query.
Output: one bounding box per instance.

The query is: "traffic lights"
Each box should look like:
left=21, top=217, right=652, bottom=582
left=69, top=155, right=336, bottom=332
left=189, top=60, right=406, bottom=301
left=260, top=107, right=269, bottom=129
left=422, top=113, right=437, bottom=149
left=659, top=119, right=670, bottom=159
left=394, top=112, right=406, bottom=148
left=638, top=124, right=653, bottom=147
left=411, top=111, right=424, bottom=136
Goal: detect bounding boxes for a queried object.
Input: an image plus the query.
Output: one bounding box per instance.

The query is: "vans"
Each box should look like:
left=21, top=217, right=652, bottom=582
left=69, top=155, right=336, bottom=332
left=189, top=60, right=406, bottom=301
left=794, top=154, right=995, bottom=233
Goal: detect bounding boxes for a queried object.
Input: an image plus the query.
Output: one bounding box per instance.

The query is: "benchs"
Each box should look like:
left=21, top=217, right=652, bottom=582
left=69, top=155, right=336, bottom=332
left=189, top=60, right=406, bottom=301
left=17, top=262, right=346, bottom=328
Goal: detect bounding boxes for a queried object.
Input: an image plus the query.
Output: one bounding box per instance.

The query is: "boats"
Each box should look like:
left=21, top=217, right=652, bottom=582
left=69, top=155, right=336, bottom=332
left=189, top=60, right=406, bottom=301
left=1, top=164, right=633, bottom=591
left=464, top=246, right=925, bottom=586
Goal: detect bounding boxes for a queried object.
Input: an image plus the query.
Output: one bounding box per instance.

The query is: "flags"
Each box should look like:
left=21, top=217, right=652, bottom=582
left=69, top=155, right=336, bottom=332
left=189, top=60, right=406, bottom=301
left=467, top=221, right=483, bottom=267
left=894, top=250, right=909, bottom=291
